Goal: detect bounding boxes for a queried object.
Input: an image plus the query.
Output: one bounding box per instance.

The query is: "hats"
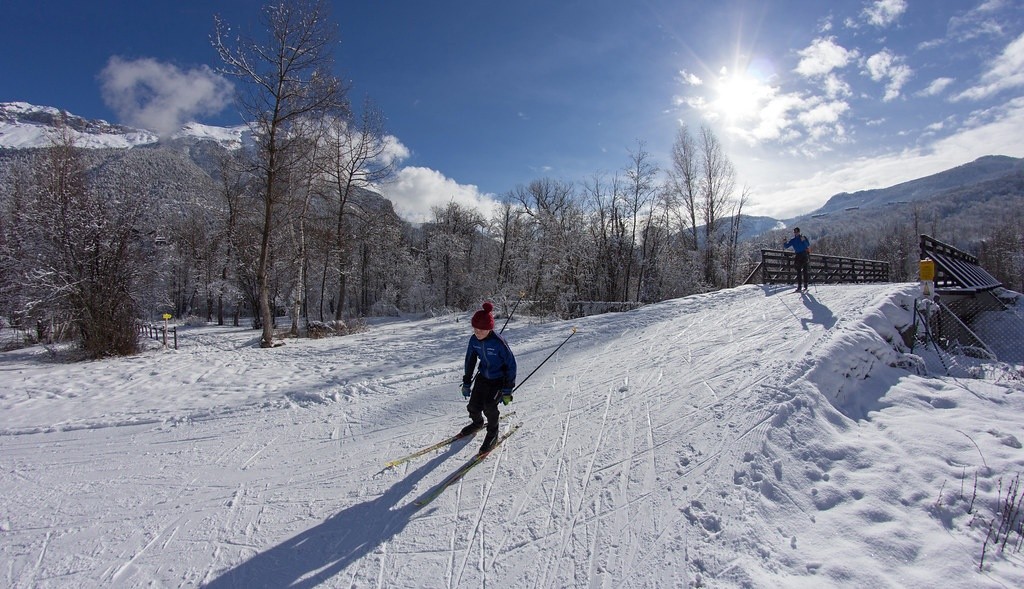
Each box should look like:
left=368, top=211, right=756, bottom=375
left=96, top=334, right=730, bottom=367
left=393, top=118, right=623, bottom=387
left=471, top=301, right=494, bottom=330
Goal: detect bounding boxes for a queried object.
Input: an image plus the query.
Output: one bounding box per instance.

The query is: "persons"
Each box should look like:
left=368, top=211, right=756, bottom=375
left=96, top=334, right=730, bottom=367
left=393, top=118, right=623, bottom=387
left=783, top=227, right=810, bottom=291
left=461, top=302, right=517, bottom=454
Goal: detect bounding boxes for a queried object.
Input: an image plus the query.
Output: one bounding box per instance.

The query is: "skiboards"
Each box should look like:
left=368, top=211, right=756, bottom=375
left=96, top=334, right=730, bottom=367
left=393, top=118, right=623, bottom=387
left=780, top=289, right=809, bottom=299
left=385, top=411, right=523, bottom=506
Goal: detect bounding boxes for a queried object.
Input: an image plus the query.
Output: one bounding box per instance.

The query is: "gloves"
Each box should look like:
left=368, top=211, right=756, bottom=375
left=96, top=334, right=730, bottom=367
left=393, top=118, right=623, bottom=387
left=459, top=383, right=471, bottom=397
left=500, top=388, right=513, bottom=406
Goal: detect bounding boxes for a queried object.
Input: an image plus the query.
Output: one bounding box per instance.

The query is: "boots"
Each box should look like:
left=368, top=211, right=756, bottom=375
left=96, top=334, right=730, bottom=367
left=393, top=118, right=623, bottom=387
left=461, top=417, right=484, bottom=435
left=479, top=423, right=499, bottom=454
